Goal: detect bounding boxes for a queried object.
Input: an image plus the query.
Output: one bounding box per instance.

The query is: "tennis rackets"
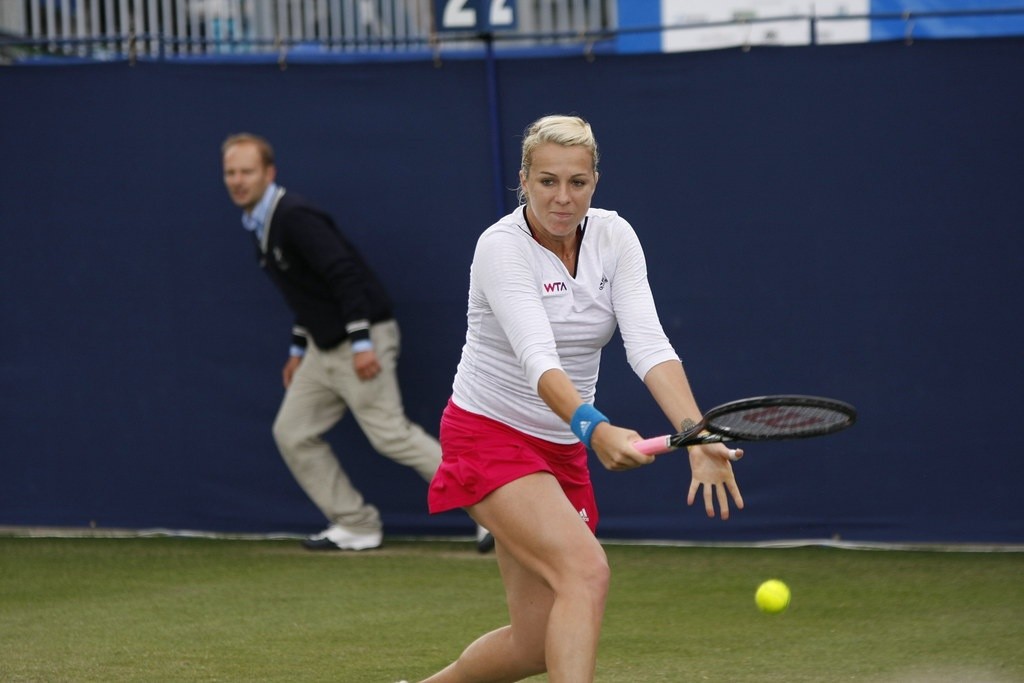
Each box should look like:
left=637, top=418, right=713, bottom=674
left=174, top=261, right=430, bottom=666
left=628, top=392, right=862, bottom=459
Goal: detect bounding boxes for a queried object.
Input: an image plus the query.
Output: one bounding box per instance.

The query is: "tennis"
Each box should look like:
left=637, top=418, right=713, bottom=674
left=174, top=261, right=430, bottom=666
left=753, top=579, right=792, bottom=615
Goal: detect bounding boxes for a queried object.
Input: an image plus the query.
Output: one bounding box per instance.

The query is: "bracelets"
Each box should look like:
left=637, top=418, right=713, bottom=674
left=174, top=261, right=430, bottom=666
left=570, top=403, right=609, bottom=450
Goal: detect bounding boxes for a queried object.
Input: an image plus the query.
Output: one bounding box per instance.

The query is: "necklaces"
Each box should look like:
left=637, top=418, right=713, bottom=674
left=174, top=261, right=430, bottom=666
left=562, top=249, right=575, bottom=261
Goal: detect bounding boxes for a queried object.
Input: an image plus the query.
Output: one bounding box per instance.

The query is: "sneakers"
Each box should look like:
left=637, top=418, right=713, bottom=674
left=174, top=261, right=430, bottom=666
left=303, top=523, right=383, bottom=550
left=476, top=521, right=494, bottom=554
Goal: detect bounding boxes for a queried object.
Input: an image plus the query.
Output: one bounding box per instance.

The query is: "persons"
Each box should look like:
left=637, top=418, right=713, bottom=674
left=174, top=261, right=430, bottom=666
left=220, top=131, right=495, bottom=554
left=414, top=113, right=745, bottom=683
